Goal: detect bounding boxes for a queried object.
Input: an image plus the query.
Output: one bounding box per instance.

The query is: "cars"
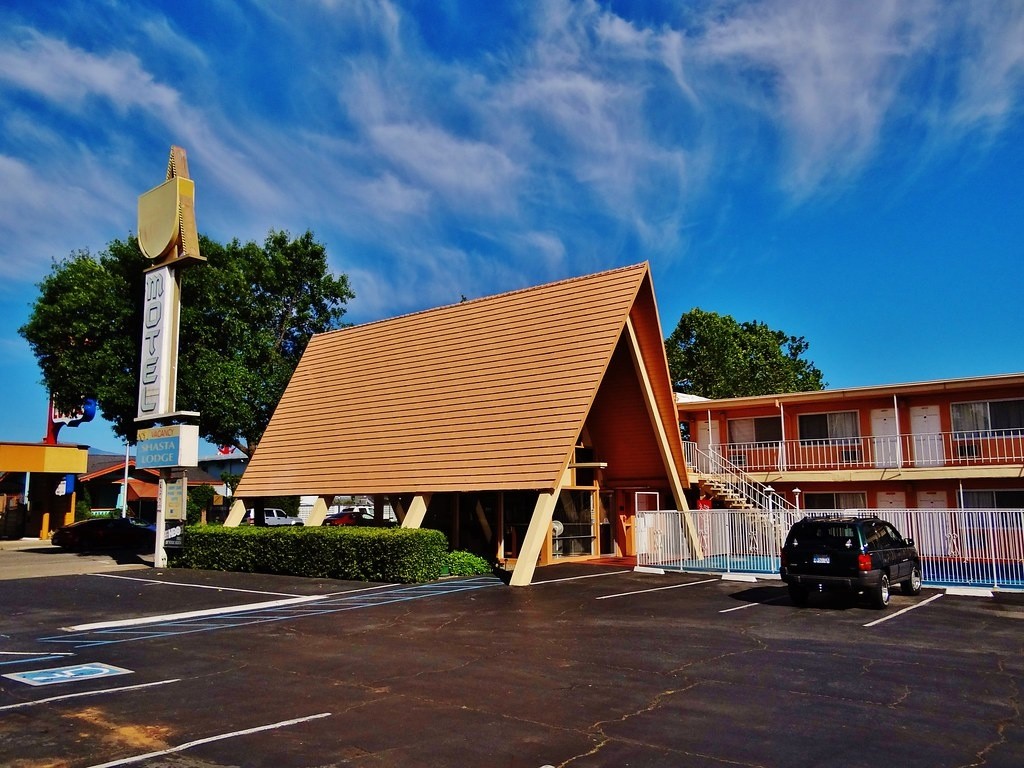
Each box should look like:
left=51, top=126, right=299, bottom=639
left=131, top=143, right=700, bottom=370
left=51, top=516, right=158, bottom=554
left=320, top=510, right=398, bottom=529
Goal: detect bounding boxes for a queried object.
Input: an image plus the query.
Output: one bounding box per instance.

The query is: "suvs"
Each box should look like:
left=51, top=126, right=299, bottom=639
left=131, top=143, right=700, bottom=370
left=780, top=513, right=925, bottom=606
left=242, top=507, right=305, bottom=527
